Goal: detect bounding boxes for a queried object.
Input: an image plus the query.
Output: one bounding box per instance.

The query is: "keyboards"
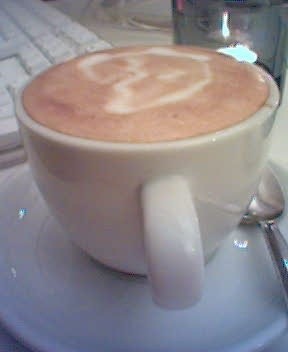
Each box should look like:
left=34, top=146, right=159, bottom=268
left=0, top=1, right=113, bottom=170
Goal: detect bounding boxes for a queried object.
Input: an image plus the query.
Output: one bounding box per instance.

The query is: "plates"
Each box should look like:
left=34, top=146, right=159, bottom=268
left=0, top=165, right=288, bottom=352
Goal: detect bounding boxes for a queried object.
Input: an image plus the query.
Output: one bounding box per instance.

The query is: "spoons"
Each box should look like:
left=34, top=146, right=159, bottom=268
left=238, top=164, right=288, bottom=302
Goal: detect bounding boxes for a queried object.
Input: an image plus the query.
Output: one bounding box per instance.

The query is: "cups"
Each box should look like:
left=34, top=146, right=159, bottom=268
left=13, top=44, right=280, bottom=310
left=170, top=2, right=287, bottom=107
